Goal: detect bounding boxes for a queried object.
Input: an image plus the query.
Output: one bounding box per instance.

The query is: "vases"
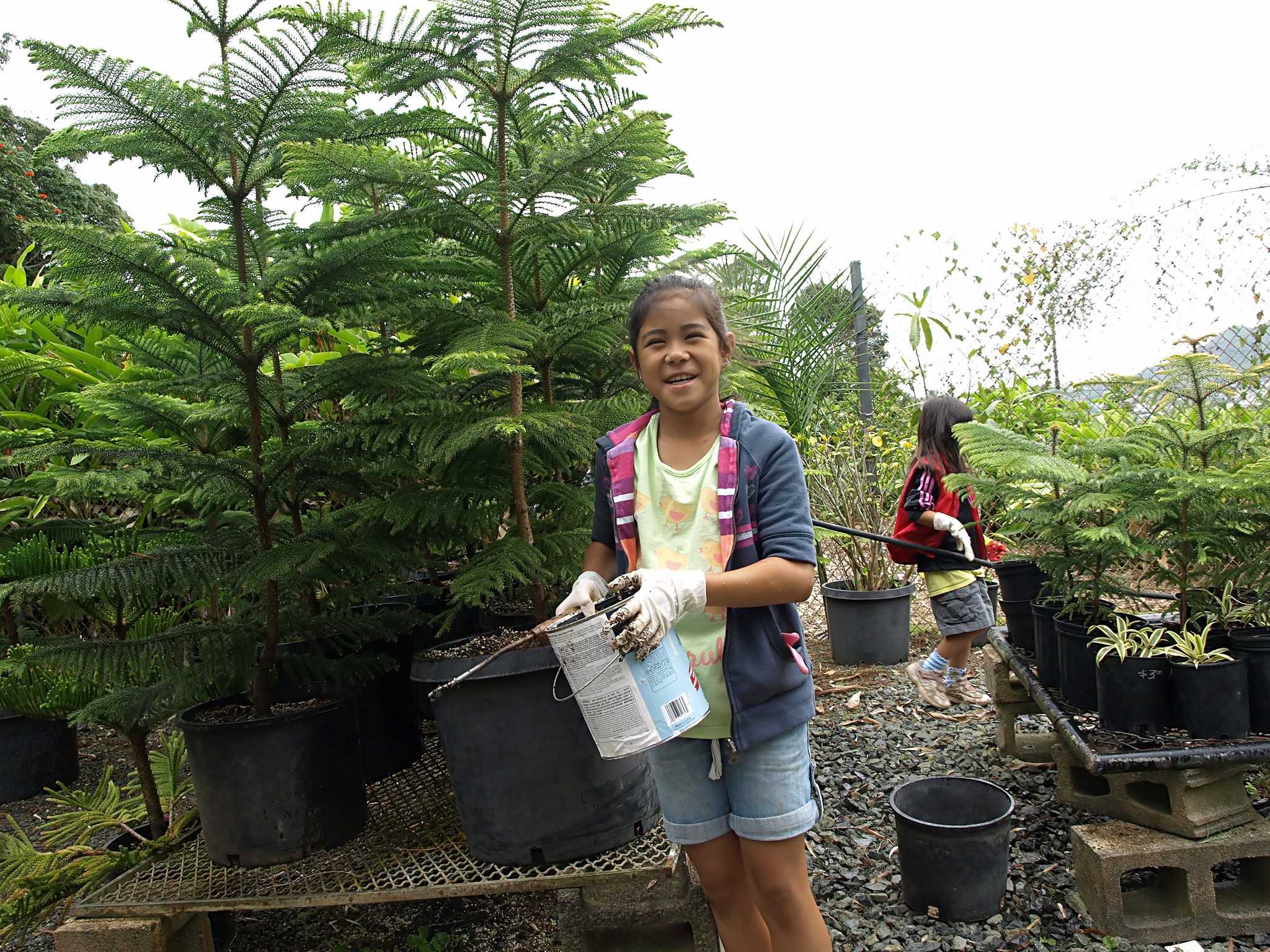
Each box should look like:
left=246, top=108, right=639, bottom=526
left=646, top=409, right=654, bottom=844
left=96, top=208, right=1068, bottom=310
left=889, top=776, right=1015, bottom=921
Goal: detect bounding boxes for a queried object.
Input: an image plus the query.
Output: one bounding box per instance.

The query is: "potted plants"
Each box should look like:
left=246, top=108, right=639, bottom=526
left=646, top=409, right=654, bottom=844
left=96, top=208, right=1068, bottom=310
left=0, top=0, right=752, bottom=864
left=788, top=325, right=1270, bottom=739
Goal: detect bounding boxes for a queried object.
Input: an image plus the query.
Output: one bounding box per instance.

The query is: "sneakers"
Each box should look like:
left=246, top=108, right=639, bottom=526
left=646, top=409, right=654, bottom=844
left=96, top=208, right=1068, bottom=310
left=943, top=677, right=992, bottom=706
left=907, top=659, right=951, bottom=708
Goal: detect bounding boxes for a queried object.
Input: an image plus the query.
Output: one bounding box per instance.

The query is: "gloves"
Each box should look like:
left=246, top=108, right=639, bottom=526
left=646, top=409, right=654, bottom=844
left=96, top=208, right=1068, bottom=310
left=607, top=568, right=707, bottom=662
left=933, top=511, right=975, bottom=561
left=555, top=571, right=609, bottom=617
left=986, top=540, right=1010, bottom=561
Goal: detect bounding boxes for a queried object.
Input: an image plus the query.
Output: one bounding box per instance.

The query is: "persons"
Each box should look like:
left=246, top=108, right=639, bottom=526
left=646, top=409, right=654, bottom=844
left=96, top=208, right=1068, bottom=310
left=556, top=276, right=832, bottom=952
left=887, top=396, right=1010, bottom=711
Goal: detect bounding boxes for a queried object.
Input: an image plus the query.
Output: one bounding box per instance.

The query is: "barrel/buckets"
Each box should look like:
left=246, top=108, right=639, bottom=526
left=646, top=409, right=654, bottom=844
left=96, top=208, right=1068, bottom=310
left=544, top=586, right=711, bottom=760
left=890, top=775, right=1017, bottom=922
